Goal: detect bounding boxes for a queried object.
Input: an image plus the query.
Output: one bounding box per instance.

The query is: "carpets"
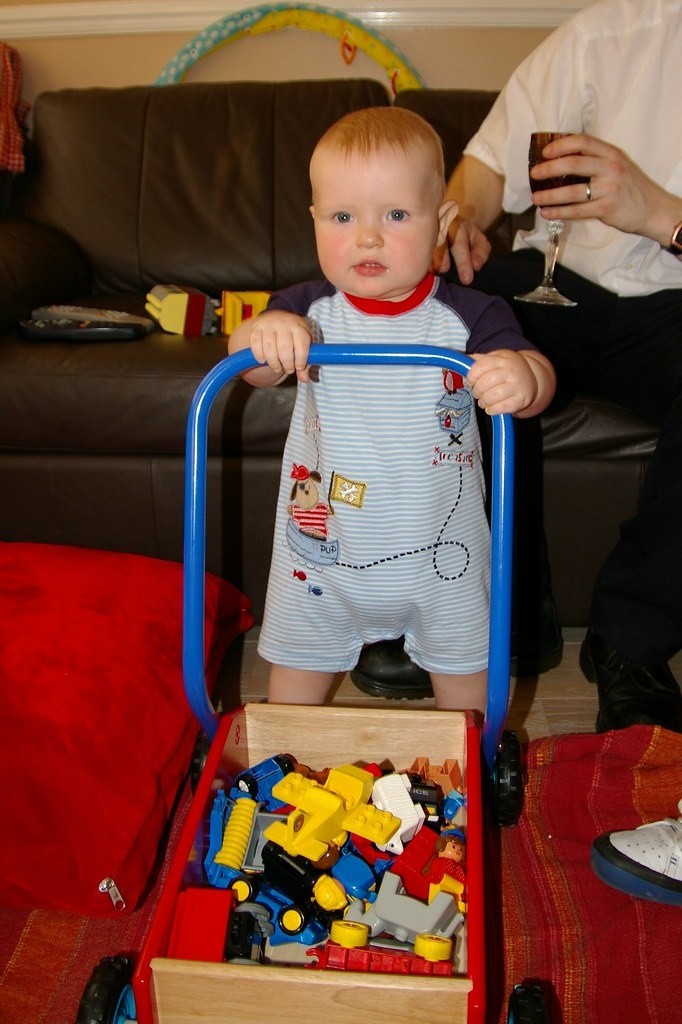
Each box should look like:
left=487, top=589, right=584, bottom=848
left=1, top=721, right=682, bottom=1023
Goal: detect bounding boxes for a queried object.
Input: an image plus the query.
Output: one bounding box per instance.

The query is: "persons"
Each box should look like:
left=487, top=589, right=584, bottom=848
left=434, top=0, right=682, bottom=733
left=229, top=107, right=558, bottom=722
left=429, top=830, right=465, bottom=899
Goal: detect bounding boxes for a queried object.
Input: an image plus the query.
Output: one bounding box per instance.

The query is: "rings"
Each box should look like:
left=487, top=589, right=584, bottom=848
left=586, top=182, right=592, bottom=202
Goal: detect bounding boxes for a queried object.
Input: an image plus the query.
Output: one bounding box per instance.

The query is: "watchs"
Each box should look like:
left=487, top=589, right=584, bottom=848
left=660, top=222, right=682, bottom=256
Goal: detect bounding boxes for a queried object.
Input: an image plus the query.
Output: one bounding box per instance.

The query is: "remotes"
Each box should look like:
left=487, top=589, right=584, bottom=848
left=19, top=304, right=157, bottom=342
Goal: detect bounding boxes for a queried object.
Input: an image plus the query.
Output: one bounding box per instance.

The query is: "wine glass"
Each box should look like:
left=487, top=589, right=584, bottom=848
left=514, top=124, right=590, bottom=308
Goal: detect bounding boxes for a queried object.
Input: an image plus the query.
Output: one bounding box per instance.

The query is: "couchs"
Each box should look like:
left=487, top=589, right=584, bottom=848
left=0, top=78, right=660, bottom=628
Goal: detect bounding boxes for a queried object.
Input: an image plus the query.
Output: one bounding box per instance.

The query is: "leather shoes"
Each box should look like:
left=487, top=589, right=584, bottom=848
left=349, top=595, right=562, bottom=701
left=578, top=629, right=681, bottom=736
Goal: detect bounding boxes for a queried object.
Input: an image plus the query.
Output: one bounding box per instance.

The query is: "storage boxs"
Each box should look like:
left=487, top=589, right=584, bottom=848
left=133, top=702, right=487, bottom=1024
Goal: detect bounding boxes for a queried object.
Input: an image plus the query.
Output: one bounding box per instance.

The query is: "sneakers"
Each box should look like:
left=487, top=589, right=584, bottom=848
left=590, top=814, right=680, bottom=908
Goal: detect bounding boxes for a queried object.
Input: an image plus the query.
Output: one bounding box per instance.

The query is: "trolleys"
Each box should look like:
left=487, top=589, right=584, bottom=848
left=71, top=341, right=565, bottom=1024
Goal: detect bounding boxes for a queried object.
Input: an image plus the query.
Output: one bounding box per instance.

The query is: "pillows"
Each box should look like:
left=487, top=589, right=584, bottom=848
left=0, top=541, right=254, bottom=923
